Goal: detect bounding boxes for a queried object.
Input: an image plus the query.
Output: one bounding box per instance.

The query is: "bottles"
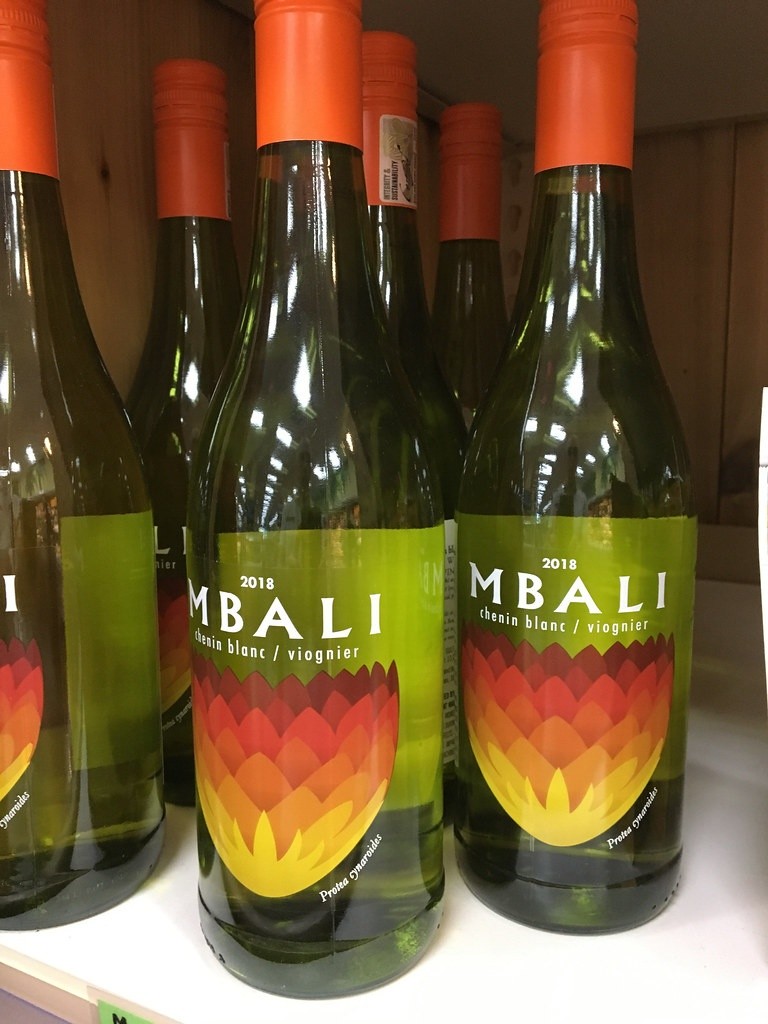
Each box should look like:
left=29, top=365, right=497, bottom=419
left=456, top=0, right=698, bottom=936
left=432, top=102, right=514, bottom=436
left=0, top=0, right=166, bottom=931
left=362, top=29, right=498, bottom=829
left=188, top=0, right=444, bottom=999
left=128, top=59, right=244, bottom=808
